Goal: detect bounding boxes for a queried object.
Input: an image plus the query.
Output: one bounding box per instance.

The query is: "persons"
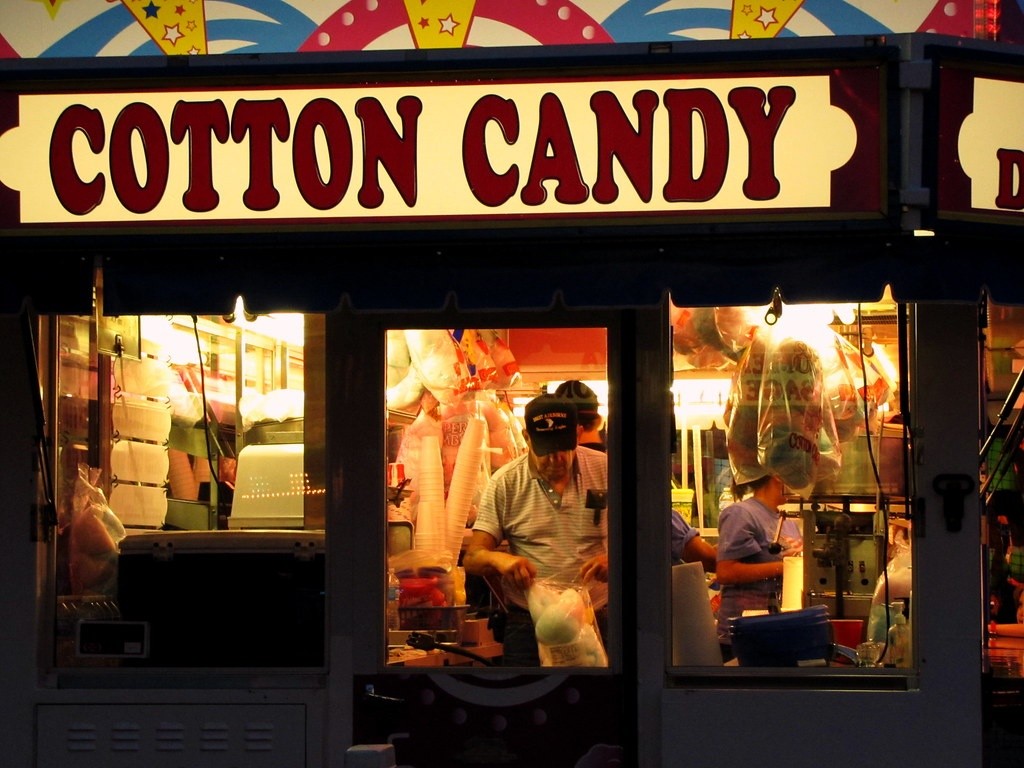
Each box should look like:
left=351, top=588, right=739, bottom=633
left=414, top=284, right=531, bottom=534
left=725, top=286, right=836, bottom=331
left=715, top=476, right=803, bottom=665
left=552, top=379, right=608, bottom=458
left=668, top=508, right=719, bottom=576
left=464, top=391, right=610, bottom=669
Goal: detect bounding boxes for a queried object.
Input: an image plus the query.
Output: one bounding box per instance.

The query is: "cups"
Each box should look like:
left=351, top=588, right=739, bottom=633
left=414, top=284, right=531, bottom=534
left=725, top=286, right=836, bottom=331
left=415, top=418, right=487, bottom=566
left=464, top=620, right=480, bottom=647
left=828, top=619, right=864, bottom=664
left=856, top=642, right=885, bottom=668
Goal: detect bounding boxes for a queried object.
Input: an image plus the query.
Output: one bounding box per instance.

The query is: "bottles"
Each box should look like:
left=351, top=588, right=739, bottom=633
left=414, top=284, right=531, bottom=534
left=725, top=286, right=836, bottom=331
left=767, top=592, right=779, bottom=615
left=719, top=488, right=735, bottom=517
left=56, top=594, right=120, bottom=668
left=887, top=614, right=913, bottom=668
left=386, top=568, right=400, bottom=629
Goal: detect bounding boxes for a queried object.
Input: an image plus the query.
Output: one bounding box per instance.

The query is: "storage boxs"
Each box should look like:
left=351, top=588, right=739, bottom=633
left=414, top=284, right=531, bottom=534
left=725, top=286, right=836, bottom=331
left=727, top=603, right=830, bottom=669
left=399, top=604, right=471, bottom=647
left=833, top=435, right=880, bottom=495
left=830, top=620, right=865, bottom=665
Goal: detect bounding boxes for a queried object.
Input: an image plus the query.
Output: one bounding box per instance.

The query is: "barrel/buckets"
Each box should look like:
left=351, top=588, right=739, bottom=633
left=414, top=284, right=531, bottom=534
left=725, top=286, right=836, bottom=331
left=671, top=489, right=694, bottom=526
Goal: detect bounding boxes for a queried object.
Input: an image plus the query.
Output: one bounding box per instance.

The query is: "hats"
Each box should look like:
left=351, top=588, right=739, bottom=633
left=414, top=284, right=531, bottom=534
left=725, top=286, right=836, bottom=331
left=525, top=394, right=579, bottom=456
left=553, top=380, right=598, bottom=414
